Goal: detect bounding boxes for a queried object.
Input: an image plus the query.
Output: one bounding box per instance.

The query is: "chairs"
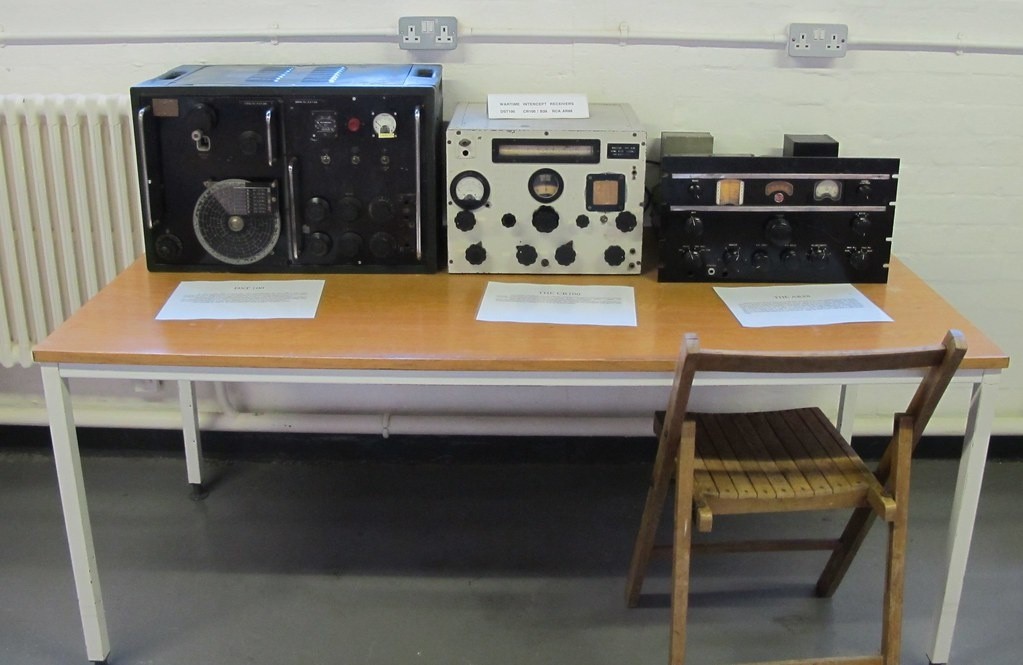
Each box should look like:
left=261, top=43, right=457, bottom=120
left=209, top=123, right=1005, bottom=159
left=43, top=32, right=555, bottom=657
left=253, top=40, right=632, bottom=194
left=626, top=327, right=966, bottom=665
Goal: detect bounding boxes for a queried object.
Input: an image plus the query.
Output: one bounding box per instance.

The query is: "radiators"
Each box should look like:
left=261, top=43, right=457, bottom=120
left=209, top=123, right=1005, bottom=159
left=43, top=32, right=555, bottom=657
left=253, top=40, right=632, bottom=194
left=0, top=95, right=146, bottom=370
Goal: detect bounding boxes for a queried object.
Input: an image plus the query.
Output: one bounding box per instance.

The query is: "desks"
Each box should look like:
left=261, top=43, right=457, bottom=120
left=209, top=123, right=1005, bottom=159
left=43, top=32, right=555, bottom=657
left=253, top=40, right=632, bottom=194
left=32, top=258, right=1009, bottom=665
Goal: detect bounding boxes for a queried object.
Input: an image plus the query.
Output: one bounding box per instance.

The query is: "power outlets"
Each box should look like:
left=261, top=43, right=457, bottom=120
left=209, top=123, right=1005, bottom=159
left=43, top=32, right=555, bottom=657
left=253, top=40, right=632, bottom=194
left=788, top=24, right=848, bottom=57
left=397, top=16, right=456, bottom=50
